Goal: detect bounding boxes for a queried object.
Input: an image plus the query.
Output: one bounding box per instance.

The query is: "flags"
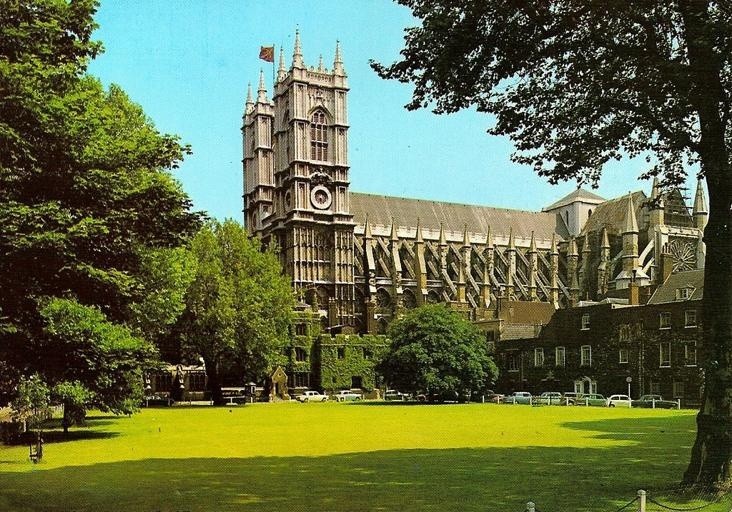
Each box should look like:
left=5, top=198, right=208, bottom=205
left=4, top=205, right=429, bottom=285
left=259, top=46, right=274, bottom=64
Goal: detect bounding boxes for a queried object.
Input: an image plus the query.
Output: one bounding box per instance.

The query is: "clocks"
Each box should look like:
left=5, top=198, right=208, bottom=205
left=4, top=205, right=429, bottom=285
left=310, top=185, right=332, bottom=209
left=284, top=189, right=292, bottom=212
left=251, top=211, right=258, bottom=230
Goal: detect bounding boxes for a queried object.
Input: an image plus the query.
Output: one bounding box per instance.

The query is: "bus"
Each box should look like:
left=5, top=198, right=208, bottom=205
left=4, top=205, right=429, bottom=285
left=221, top=387, right=264, bottom=401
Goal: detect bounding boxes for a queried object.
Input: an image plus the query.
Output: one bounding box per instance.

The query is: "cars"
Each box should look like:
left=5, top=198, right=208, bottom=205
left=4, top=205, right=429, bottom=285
left=141, top=394, right=174, bottom=407
left=636, top=394, right=663, bottom=401
left=606, top=394, right=635, bottom=408
left=487, top=389, right=606, bottom=407
left=332, top=388, right=363, bottom=402
left=382, top=388, right=470, bottom=402
left=295, top=391, right=329, bottom=402
